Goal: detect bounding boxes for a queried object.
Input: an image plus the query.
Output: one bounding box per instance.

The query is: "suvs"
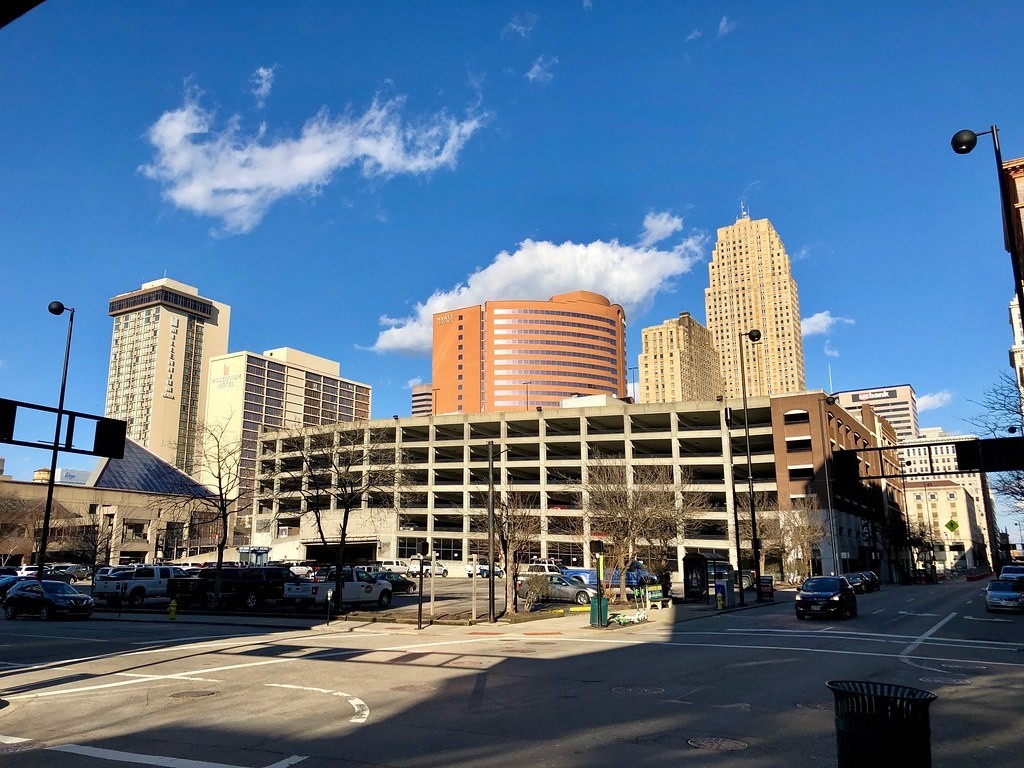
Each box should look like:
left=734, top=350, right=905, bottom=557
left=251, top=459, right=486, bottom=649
left=285, top=563, right=314, bottom=577
left=795, top=575, right=858, bottom=621
left=16, top=564, right=93, bottom=580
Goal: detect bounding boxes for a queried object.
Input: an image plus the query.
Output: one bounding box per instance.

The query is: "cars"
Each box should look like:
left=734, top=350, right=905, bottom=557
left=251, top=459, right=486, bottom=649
left=981, top=579, right=1024, bottom=613
left=518, top=574, right=598, bottom=605
left=315, top=568, right=334, bottom=581
left=2, top=580, right=95, bottom=622
left=370, top=571, right=417, bottom=594
left=27, top=570, right=78, bottom=585
left=999, top=565, right=1024, bottom=580
left=838, top=571, right=880, bottom=595
left=128, top=562, right=249, bottom=570
left=409, top=560, right=448, bottom=577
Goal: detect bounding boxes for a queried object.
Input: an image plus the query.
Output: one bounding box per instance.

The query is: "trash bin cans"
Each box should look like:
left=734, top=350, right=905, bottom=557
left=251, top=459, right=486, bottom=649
left=590, top=596, right=609, bottom=626
left=827, top=681, right=938, bottom=768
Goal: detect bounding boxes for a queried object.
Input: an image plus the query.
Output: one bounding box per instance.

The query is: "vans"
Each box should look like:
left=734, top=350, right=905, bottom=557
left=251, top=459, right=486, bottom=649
left=528, top=564, right=561, bottom=576
left=534, top=558, right=570, bottom=572
left=355, top=566, right=379, bottom=579
left=613, top=560, right=646, bottom=587
left=96, top=567, right=135, bottom=577
left=382, top=560, right=409, bottom=574
left=466, top=557, right=503, bottom=578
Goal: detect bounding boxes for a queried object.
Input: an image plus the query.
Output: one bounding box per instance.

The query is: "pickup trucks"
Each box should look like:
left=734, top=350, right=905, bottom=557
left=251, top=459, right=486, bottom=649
left=284, top=569, right=393, bottom=610
left=93, top=566, right=195, bottom=609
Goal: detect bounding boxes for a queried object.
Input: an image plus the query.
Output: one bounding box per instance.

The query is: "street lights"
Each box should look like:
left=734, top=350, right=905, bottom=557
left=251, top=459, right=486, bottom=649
left=36, top=300, right=75, bottom=578
